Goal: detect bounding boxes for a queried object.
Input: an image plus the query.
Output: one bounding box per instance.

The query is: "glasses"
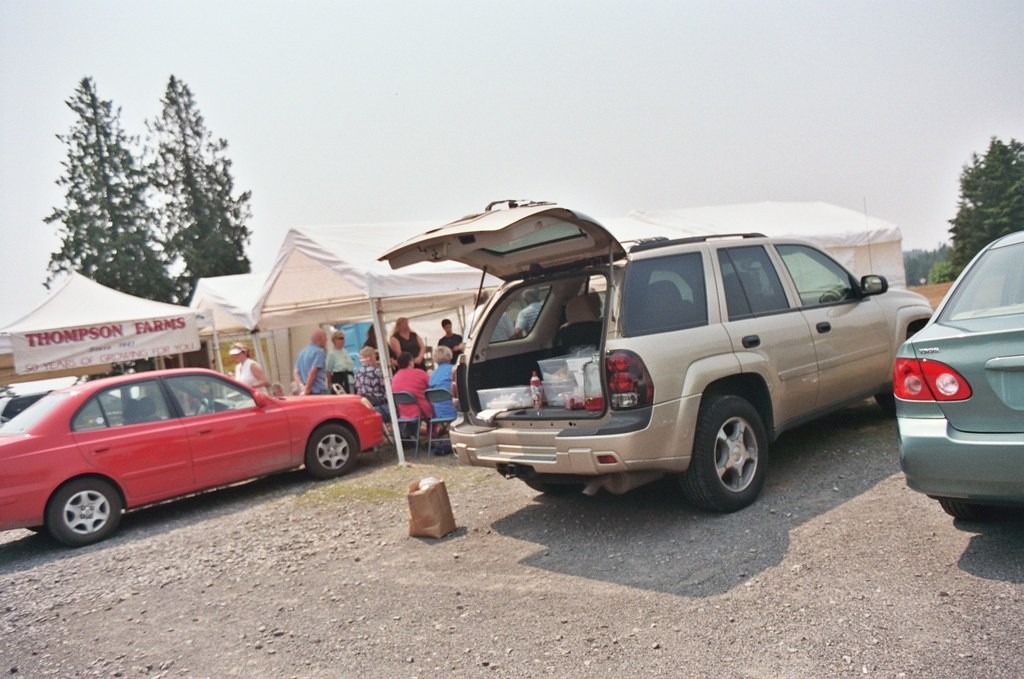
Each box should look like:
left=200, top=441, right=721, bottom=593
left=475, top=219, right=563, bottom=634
left=335, top=337, right=345, bottom=340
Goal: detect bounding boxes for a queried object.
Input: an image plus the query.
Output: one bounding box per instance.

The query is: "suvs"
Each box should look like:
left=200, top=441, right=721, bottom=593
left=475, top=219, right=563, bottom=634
left=376, top=199, right=937, bottom=514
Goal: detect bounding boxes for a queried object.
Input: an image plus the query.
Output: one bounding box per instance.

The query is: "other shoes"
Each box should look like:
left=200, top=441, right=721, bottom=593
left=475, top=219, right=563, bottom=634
left=432, top=422, right=450, bottom=438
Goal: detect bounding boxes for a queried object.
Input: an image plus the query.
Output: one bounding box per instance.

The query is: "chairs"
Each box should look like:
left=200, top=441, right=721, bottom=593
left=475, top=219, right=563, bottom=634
left=365, top=396, right=392, bottom=451
left=392, top=390, right=426, bottom=459
left=139, top=397, right=160, bottom=422
left=424, top=388, right=452, bottom=459
left=724, top=271, right=758, bottom=313
left=558, top=292, right=602, bottom=348
left=646, top=279, right=684, bottom=324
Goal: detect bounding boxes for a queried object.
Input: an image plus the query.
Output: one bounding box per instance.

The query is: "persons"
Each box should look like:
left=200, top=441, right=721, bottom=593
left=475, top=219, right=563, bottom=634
left=327, top=331, right=354, bottom=395
left=429, top=345, right=457, bottom=436
left=391, top=352, right=433, bottom=439
left=228, top=342, right=268, bottom=396
left=354, top=346, right=392, bottom=432
left=363, top=325, right=396, bottom=373
left=515, top=288, right=543, bottom=339
left=438, top=319, right=464, bottom=364
left=390, top=318, right=428, bottom=371
left=293, top=328, right=328, bottom=395
left=462, top=290, right=514, bottom=345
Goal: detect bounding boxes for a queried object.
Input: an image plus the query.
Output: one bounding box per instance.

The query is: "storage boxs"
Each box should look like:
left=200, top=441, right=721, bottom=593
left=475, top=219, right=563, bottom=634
left=476, top=386, right=533, bottom=411
left=538, top=353, right=592, bottom=406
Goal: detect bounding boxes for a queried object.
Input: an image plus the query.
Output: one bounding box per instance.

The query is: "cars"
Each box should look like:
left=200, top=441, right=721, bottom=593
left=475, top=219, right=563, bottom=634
left=893, top=230, right=1024, bottom=523
left=0, top=366, right=384, bottom=547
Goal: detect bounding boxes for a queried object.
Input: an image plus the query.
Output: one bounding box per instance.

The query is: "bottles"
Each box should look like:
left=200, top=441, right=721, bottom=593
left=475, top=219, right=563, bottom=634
left=530, top=371, right=542, bottom=409
left=582, top=353, right=604, bottom=410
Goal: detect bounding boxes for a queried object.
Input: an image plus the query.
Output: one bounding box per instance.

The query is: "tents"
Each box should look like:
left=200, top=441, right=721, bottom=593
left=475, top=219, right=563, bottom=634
left=0, top=271, right=226, bottom=399
left=188, top=199, right=908, bottom=467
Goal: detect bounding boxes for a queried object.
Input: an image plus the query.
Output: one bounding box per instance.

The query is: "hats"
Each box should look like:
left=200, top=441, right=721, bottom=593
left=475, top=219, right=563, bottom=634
left=229, top=343, right=248, bottom=355
left=358, top=346, right=374, bottom=357
